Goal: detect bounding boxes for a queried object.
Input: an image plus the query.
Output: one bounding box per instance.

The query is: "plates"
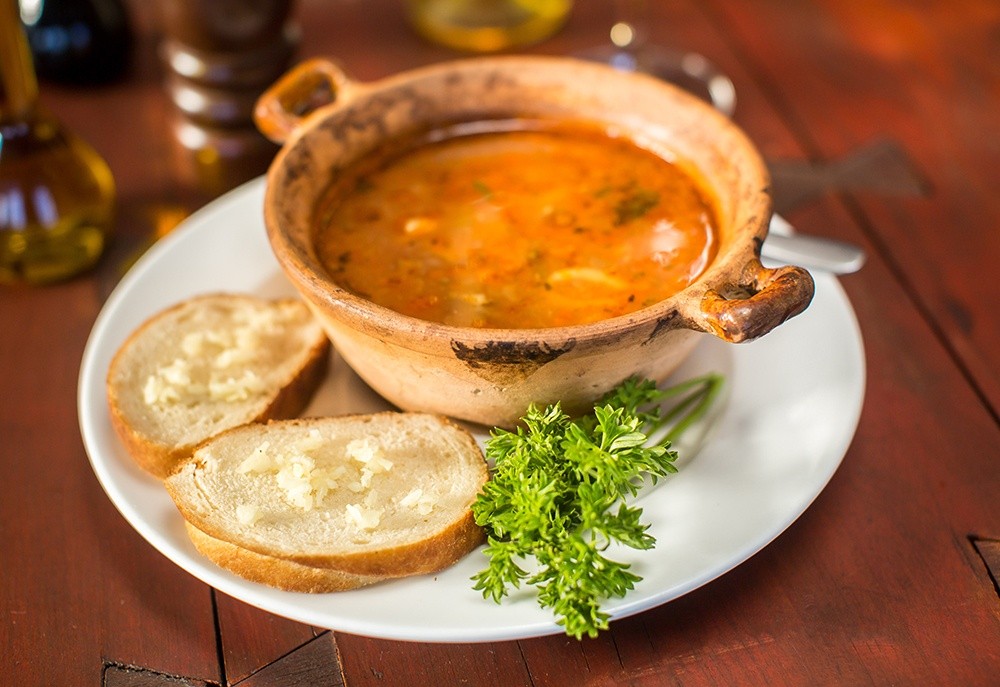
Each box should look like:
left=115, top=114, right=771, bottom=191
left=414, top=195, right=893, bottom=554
left=74, top=166, right=869, bottom=641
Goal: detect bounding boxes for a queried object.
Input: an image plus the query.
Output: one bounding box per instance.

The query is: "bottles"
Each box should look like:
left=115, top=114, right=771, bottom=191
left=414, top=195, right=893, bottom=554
left=18, top=0, right=140, bottom=95
left=0, top=108, right=117, bottom=285
left=401, top=0, right=572, bottom=54
left=143, top=2, right=313, bottom=220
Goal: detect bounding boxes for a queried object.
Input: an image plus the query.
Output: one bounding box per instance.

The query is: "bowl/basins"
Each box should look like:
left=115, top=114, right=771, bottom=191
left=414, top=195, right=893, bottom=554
left=253, top=54, right=816, bottom=435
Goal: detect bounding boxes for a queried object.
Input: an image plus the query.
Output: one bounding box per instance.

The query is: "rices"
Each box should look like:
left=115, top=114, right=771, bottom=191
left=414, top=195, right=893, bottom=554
left=143, top=303, right=437, bottom=530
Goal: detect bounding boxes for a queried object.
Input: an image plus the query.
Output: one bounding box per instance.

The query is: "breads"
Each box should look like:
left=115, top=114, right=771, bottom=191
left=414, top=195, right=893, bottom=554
left=106, top=293, right=331, bottom=475
left=164, top=410, right=490, bottom=595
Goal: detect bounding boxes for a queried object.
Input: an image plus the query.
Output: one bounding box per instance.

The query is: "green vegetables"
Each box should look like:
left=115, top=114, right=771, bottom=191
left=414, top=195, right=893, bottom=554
left=470, top=371, right=720, bottom=640
